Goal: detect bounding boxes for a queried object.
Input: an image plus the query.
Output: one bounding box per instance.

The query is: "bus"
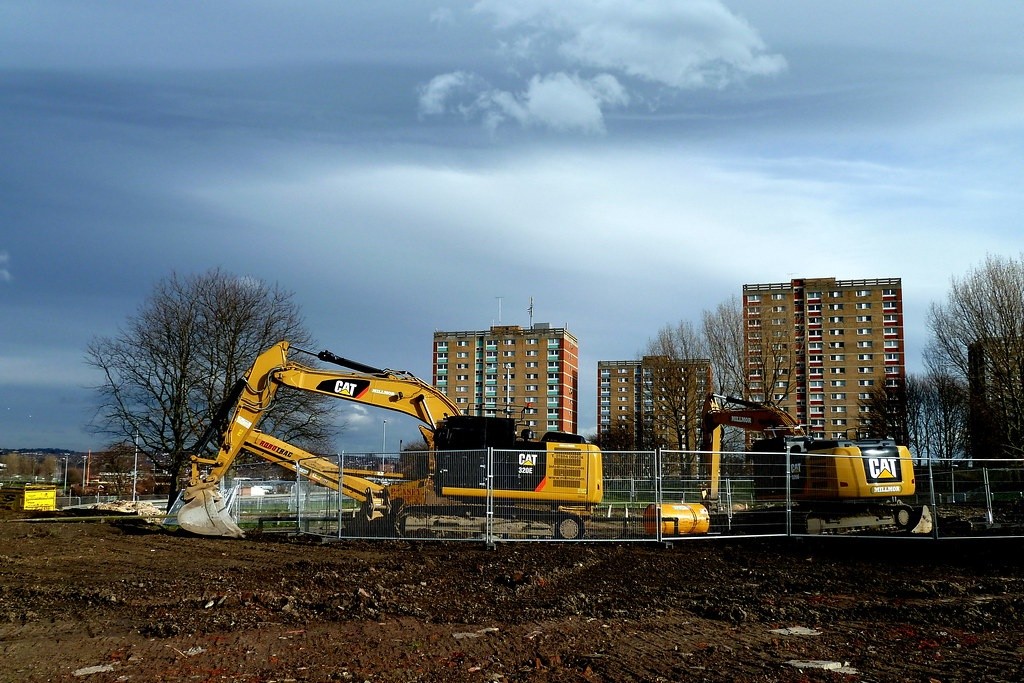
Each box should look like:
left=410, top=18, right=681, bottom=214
left=98, top=473, right=172, bottom=496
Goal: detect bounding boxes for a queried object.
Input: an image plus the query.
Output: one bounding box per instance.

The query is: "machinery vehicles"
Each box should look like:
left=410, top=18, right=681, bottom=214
left=700, top=392, right=934, bottom=536
left=160, top=341, right=603, bottom=544
